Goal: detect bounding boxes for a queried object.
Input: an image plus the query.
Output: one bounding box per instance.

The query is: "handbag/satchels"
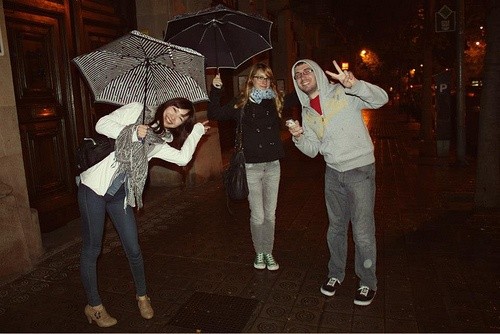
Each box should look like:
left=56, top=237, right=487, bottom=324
left=225, top=149, right=249, bottom=200
left=77, top=134, right=111, bottom=173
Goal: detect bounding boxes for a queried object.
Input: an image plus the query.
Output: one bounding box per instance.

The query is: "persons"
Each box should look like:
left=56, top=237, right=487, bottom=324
left=78, top=97, right=210, bottom=327
left=288, top=58, right=389, bottom=306
left=207, top=62, right=301, bottom=271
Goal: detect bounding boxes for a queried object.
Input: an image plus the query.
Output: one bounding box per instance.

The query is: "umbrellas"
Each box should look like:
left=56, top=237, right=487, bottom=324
left=72, top=29, right=210, bottom=143
left=163, top=4, right=273, bottom=87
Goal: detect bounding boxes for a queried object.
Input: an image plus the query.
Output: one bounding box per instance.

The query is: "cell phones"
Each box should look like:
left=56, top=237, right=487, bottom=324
left=286, top=119, right=296, bottom=127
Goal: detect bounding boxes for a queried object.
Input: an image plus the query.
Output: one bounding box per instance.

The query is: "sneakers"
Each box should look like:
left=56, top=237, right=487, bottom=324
left=354, top=286, right=376, bottom=306
left=254, top=252, right=266, bottom=269
left=321, top=277, right=341, bottom=297
left=264, top=254, right=279, bottom=271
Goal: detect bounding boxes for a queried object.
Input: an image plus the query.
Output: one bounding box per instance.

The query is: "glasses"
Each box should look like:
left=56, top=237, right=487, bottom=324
left=253, top=76, right=270, bottom=81
left=294, top=68, right=313, bottom=80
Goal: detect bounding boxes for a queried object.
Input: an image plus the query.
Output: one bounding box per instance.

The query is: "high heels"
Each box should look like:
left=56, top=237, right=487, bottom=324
left=85, top=304, right=117, bottom=327
left=136, top=295, right=154, bottom=319
